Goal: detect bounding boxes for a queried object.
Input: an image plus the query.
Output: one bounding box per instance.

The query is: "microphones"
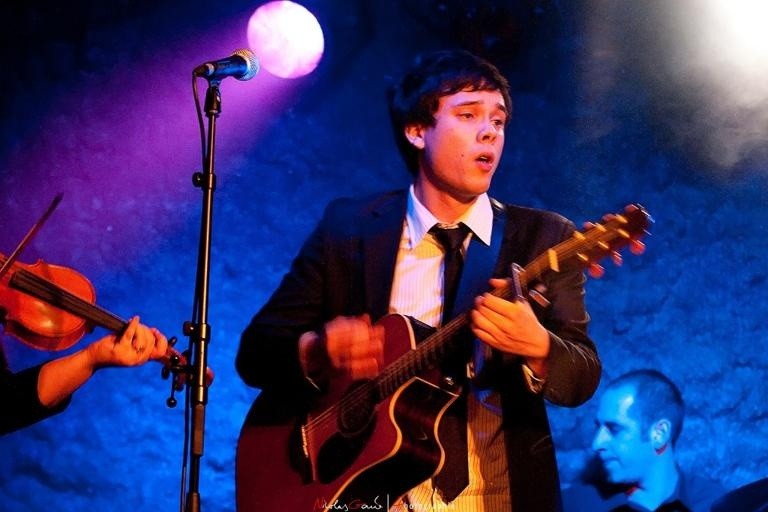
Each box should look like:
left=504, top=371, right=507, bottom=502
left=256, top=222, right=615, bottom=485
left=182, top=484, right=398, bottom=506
left=193, top=48, right=260, bottom=81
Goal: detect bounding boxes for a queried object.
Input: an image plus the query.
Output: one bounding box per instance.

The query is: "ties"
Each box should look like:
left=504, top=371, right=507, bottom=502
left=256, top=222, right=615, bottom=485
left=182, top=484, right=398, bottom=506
left=427, top=222, right=471, bottom=502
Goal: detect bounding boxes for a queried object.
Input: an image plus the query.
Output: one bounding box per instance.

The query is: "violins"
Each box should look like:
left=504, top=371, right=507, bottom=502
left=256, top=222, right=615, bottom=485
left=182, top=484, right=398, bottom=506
left=0, top=253, right=215, bottom=408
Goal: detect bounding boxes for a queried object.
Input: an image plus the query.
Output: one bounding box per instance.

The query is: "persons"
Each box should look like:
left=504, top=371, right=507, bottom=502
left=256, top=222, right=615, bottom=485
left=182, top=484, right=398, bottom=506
left=233, top=47, right=603, bottom=511
left=562, top=367, right=732, bottom=511
left=1, top=313, right=170, bottom=440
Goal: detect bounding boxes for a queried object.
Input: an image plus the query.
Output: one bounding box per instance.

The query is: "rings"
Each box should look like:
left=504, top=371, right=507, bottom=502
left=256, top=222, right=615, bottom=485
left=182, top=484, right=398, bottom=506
left=131, top=342, right=146, bottom=354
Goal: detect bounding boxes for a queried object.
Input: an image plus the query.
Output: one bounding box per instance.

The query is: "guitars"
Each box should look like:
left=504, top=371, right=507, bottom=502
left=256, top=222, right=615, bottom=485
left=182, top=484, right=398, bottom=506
left=234, top=203, right=655, bottom=512
